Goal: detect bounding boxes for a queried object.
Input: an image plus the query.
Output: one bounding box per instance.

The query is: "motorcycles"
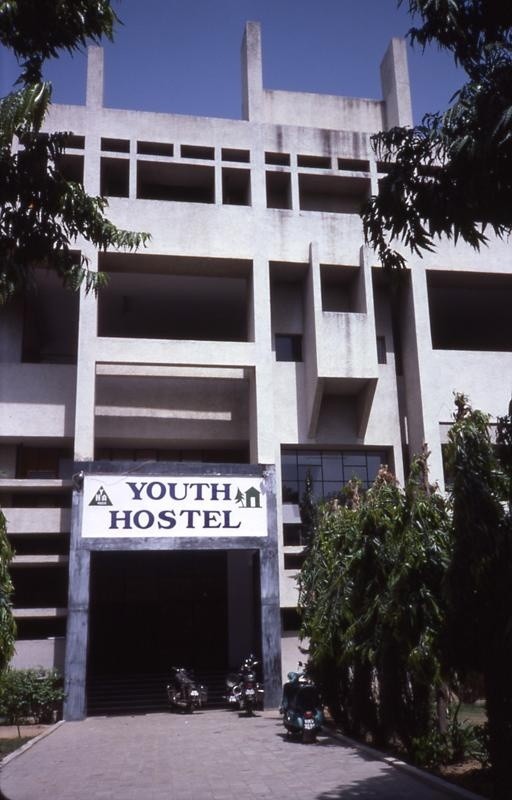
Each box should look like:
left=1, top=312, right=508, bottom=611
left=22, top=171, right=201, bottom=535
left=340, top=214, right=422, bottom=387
left=228, top=653, right=265, bottom=715
left=274, top=658, right=326, bottom=745
left=164, top=664, right=209, bottom=713
